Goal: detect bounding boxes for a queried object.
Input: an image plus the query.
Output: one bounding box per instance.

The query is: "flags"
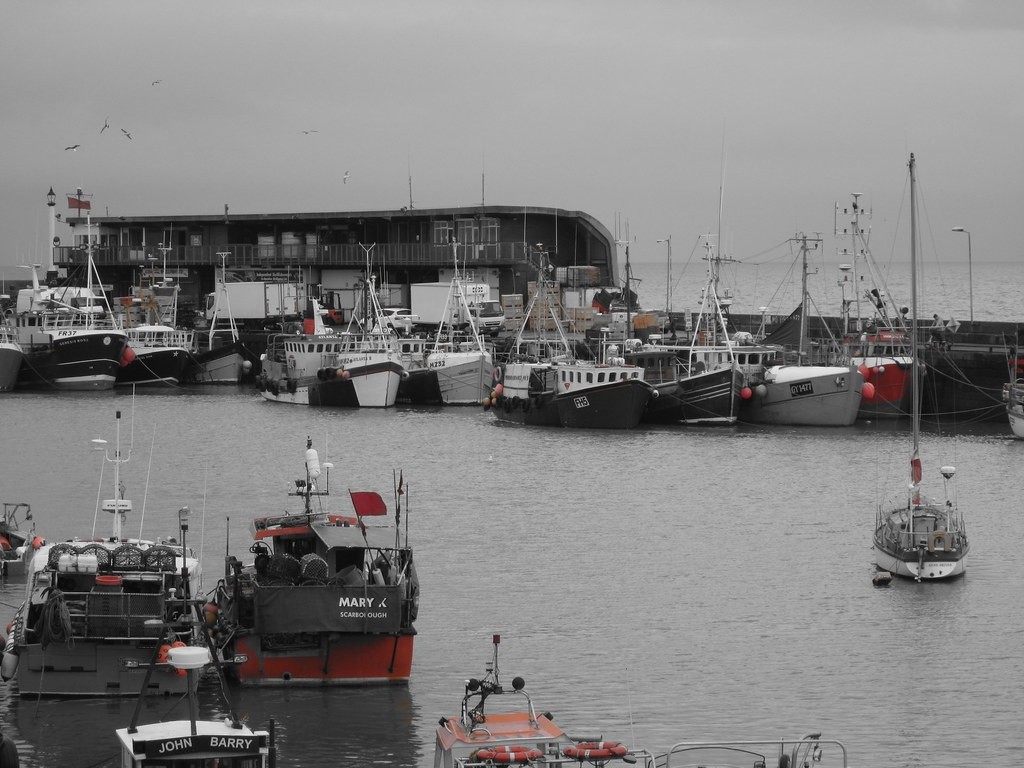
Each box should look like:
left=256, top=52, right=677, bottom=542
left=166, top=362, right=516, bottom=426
left=910, top=448, right=922, bottom=486
left=911, top=491, right=920, bottom=504
left=350, top=491, right=387, bottom=516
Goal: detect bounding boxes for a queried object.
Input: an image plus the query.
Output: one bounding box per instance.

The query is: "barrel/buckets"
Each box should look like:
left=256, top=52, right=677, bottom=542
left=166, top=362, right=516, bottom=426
left=89, top=576, right=123, bottom=642
left=339, top=565, right=368, bottom=585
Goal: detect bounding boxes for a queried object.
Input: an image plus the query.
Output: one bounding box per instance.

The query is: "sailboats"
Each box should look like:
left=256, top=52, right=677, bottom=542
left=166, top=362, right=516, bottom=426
left=868, top=152, right=971, bottom=582
left=831, top=190, right=919, bottom=420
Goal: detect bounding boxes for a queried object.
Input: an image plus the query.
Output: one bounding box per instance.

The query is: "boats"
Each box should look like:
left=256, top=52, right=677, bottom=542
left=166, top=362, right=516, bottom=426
left=257, top=242, right=405, bottom=409
left=197, top=251, right=263, bottom=386
left=113, top=507, right=277, bottom=768
left=430, top=633, right=659, bottom=768
left=689, top=185, right=864, bottom=427
left=1, top=186, right=129, bottom=393
left=621, top=229, right=751, bottom=426
left=204, top=436, right=422, bottom=689
left=398, top=237, right=496, bottom=406
left=122, top=224, right=200, bottom=387
left=479, top=201, right=660, bottom=430
left=0, top=382, right=208, bottom=701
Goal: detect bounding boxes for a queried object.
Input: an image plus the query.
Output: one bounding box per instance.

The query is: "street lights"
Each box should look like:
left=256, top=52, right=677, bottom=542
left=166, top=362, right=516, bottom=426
left=951, top=227, right=973, bottom=334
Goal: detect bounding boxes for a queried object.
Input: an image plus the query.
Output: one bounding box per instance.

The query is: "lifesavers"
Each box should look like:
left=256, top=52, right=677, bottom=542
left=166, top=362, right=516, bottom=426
left=563, top=741, right=629, bottom=758
left=494, top=367, right=503, bottom=382
left=475, top=745, right=543, bottom=762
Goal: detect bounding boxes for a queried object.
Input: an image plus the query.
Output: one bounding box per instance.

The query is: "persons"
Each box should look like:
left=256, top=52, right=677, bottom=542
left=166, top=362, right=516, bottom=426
left=929, top=314, right=947, bottom=348
left=776, top=754, right=792, bottom=768
